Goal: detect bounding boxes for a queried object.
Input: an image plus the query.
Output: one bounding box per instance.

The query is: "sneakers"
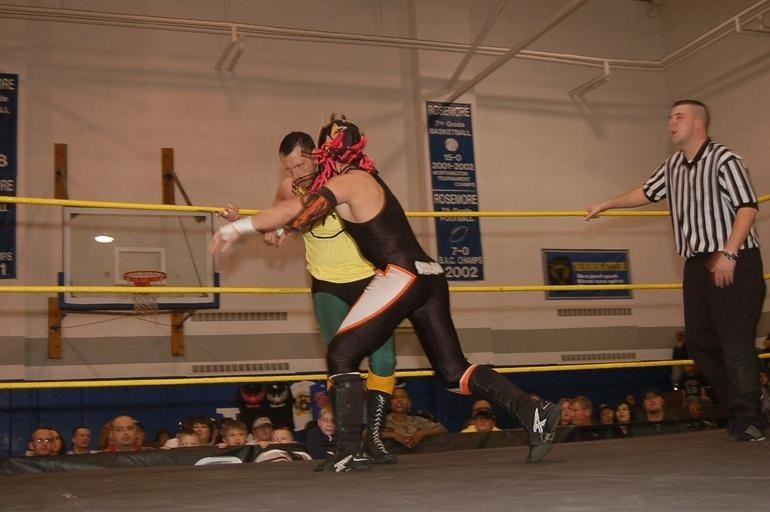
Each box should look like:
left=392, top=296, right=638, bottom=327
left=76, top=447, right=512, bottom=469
left=736, top=423, right=765, bottom=441
left=685, top=415, right=718, bottom=431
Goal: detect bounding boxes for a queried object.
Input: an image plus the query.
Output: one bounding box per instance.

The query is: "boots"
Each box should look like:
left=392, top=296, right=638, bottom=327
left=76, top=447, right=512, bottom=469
left=313, top=370, right=396, bottom=473
left=467, top=364, right=561, bottom=463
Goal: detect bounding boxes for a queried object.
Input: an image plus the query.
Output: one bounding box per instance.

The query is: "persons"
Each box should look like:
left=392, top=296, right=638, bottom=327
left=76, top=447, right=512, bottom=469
left=460, top=399, right=501, bottom=433
left=529, top=386, right=727, bottom=428
left=207, top=116, right=562, bottom=469
left=158, top=381, right=338, bottom=448
left=27, top=415, right=155, bottom=457
left=585, top=99, right=766, bottom=440
left=360, top=380, right=447, bottom=449
left=215, top=132, right=395, bottom=465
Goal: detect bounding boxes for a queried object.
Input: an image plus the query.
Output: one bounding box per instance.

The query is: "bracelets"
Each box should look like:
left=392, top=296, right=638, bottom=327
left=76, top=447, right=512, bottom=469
left=721, top=249, right=740, bottom=261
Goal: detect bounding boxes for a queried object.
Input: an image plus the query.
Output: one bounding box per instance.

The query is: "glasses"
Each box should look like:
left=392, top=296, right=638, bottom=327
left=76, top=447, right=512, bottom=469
left=35, top=438, right=52, bottom=444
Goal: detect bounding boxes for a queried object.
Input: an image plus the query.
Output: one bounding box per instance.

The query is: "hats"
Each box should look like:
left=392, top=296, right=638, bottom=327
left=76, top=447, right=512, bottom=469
left=472, top=399, right=492, bottom=409
left=252, top=417, right=271, bottom=428
